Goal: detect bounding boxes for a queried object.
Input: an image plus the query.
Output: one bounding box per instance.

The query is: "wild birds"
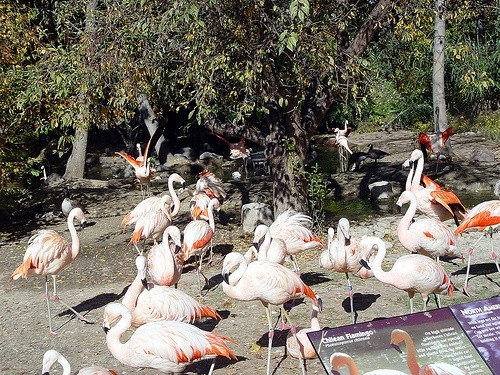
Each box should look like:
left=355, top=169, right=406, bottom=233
left=11, top=122, right=500, bottom=336
left=390, top=328, right=467, bottom=375
left=220, top=252, right=321, bottom=375
left=42, top=350, right=117, bottom=374
left=101, top=301, right=239, bottom=375
left=286, top=294, right=322, bottom=359
left=329, top=351, right=406, bottom=375
